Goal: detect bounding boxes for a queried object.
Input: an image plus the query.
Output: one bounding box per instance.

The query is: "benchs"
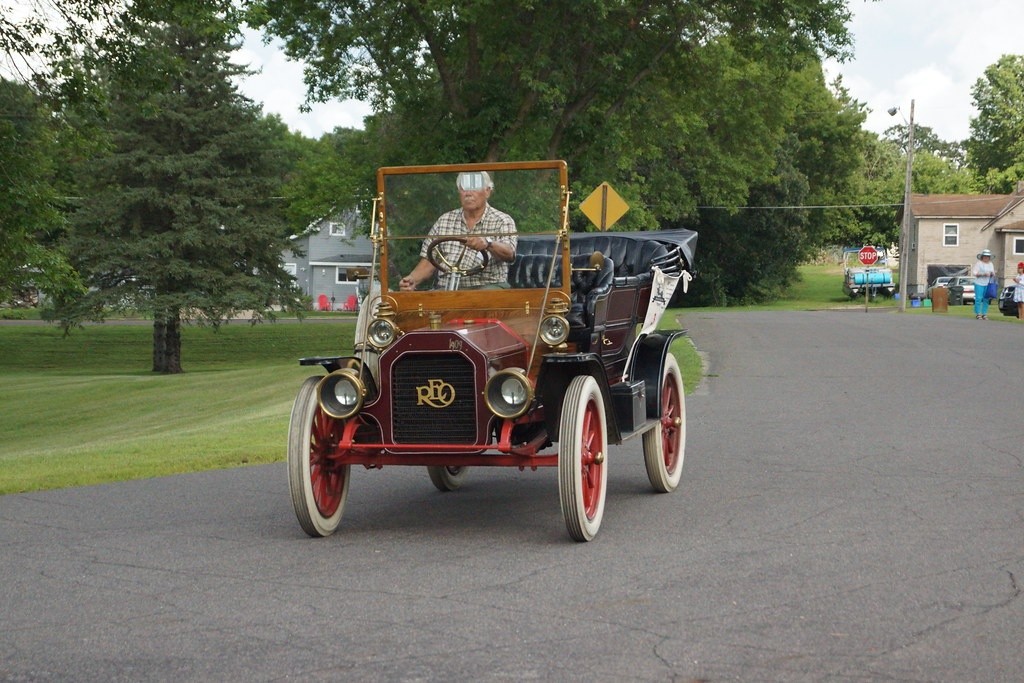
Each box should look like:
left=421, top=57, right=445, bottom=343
left=512, top=233, right=669, bottom=288
left=506, top=253, right=614, bottom=348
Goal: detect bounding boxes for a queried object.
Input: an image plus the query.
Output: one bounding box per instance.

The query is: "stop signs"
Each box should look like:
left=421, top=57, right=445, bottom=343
left=858, top=246, right=878, bottom=266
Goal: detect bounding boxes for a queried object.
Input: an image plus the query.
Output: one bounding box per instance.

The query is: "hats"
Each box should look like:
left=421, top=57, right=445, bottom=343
left=1018, top=262, right=1024, bottom=268
left=983, top=249, right=991, bottom=255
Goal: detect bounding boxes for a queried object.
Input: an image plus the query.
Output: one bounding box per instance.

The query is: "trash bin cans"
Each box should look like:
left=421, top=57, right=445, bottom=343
left=932, top=288, right=948, bottom=313
left=948, top=286, right=964, bottom=305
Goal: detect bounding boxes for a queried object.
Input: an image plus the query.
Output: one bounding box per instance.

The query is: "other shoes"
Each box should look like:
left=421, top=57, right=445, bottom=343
left=982, top=315, right=988, bottom=320
left=976, top=314, right=982, bottom=320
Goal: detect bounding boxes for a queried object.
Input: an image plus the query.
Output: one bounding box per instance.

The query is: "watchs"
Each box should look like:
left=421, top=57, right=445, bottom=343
left=485, top=236, right=492, bottom=249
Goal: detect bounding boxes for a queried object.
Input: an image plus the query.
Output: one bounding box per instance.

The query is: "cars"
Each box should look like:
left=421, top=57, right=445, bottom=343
left=287, top=162, right=699, bottom=543
left=943, top=276, right=975, bottom=305
left=998, top=285, right=1018, bottom=318
left=926, top=277, right=953, bottom=299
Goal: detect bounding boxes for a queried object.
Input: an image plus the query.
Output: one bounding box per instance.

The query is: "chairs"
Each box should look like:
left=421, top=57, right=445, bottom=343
left=343, top=295, right=356, bottom=312
left=318, top=295, right=331, bottom=311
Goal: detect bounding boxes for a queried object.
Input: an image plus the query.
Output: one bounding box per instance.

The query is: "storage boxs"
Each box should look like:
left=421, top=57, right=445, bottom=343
left=911, top=300, right=920, bottom=307
left=923, top=298, right=931, bottom=306
left=894, top=294, right=900, bottom=300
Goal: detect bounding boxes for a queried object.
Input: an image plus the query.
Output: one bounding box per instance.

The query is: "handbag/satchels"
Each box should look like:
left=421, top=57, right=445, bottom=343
left=984, top=273, right=998, bottom=298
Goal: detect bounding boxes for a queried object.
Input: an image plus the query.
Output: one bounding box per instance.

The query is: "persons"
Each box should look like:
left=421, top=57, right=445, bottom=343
left=399, top=171, right=518, bottom=291
left=972, top=250, right=997, bottom=321
left=1013, top=262, right=1024, bottom=320
